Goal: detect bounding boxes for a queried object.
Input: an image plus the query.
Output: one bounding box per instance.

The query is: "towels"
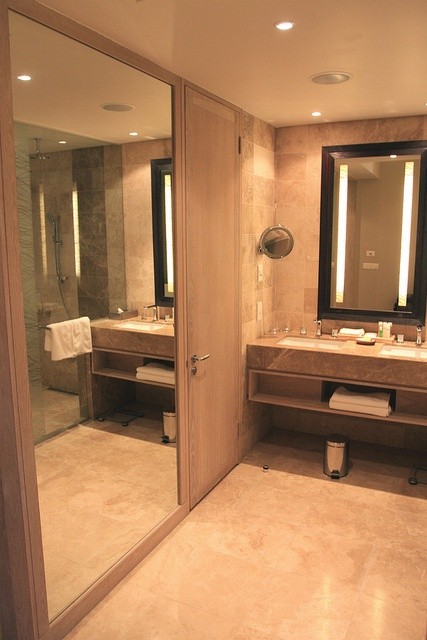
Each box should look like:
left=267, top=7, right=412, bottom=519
left=329, top=385, right=394, bottom=419
left=44, top=317, right=93, bottom=363
left=135, top=361, right=175, bottom=387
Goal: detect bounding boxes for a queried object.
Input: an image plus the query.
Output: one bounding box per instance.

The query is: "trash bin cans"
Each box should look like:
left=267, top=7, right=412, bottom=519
left=322, top=433, right=349, bottom=479
left=160, top=410, right=176, bottom=443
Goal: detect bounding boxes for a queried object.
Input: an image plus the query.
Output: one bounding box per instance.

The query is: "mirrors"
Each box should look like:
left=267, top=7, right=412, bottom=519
left=150, top=156, right=174, bottom=307
left=316, top=141, right=427, bottom=327
left=0, top=0, right=189, bottom=640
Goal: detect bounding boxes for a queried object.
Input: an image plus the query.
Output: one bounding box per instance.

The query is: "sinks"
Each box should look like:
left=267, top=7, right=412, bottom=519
left=111, top=320, right=163, bottom=331
left=378, top=342, right=427, bottom=359
left=275, top=334, right=348, bottom=351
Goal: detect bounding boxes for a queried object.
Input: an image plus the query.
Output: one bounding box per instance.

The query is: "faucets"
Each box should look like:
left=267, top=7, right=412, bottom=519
left=144, top=304, right=159, bottom=321
left=314, top=319, right=322, bottom=336
left=415, top=324, right=422, bottom=345
